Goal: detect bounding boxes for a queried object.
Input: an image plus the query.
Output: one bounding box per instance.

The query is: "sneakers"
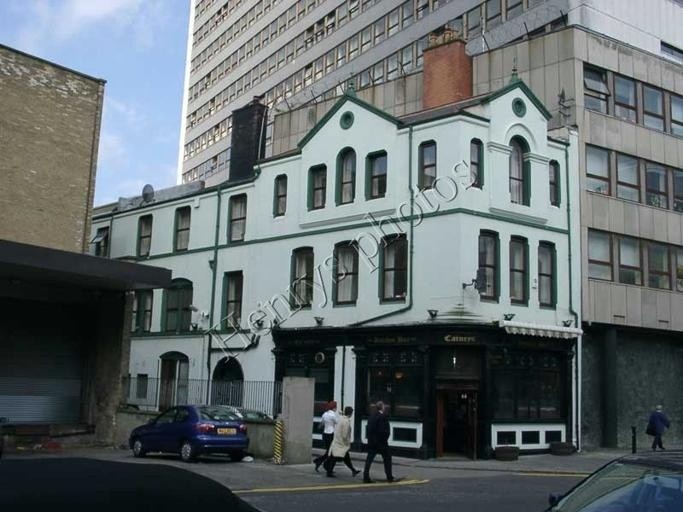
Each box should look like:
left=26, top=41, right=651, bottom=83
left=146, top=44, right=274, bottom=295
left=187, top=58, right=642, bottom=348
left=352, top=470, right=361, bottom=477
left=327, top=474, right=335, bottom=477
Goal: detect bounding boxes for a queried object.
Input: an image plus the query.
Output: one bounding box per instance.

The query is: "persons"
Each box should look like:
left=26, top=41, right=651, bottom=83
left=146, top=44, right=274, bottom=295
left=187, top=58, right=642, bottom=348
left=313, top=401, right=342, bottom=472
left=325, top=407, right=361, bottom=477
left=362, top=401, right=400, bottom=483
left=648, top=404, right=670, bottom=451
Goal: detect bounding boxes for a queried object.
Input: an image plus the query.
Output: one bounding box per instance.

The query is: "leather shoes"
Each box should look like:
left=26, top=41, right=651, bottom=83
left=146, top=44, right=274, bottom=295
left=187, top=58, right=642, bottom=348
left=389, top=478, right=399, bottom=483
left=365, top=480, right=376, bottom=483
left=313, top=459, right=320, bottom=472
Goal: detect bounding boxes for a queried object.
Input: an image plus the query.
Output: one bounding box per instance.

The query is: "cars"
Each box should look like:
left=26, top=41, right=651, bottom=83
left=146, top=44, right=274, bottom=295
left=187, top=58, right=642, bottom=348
left=128, top=403, right=251, bottom=463
left=538, top=450, right=683, bottom=512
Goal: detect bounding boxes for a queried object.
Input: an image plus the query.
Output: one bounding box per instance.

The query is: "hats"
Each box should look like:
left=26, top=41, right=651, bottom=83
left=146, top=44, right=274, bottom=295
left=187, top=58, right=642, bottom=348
left=327, top=401, right=337, bottom=410
